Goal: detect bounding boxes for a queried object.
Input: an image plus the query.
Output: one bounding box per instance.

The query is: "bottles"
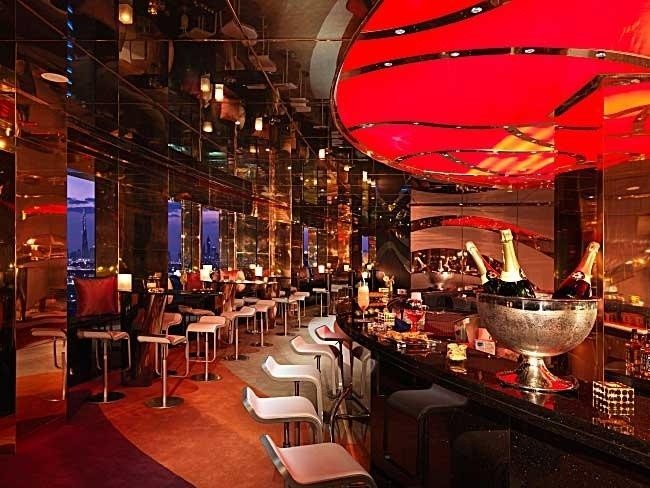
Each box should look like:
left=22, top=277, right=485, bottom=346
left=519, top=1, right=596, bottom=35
left=546, top=239, right=602, bottom=308
left=464, top=238, right=500, bottom=292
left=416, top=256, right=450, bottom=271
left=496, top=227, right=539, bottom=309
left=626, top=327, right=650, bottom=379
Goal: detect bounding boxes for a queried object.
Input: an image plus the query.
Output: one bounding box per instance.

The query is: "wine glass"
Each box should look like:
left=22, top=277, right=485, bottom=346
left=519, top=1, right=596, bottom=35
left=356, top=286, right=371, bottom=322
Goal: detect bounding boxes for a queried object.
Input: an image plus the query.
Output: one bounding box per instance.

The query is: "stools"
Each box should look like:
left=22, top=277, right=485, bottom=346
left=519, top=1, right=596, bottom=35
left=154, top=312, right=182, bottom=377
left=259, top=353, right=326, bottom=447
left=136, top=333, right=191, bottom=410
left=287, top=335, right=341, bottom=397
left=245, top=299, right=273, bottom=348
left=183, top=314, right=227, bottom=384
left=240, top=283, right=359, bottom=338
left=79, top=330, right=132, bottom=406
left=178, top=304, right=215, bottom=362
left=217, top=305, right=256, bottom=361
left=257, top=431, right=374, bottom=487
left=240, top=384, right=325, bottom=453
